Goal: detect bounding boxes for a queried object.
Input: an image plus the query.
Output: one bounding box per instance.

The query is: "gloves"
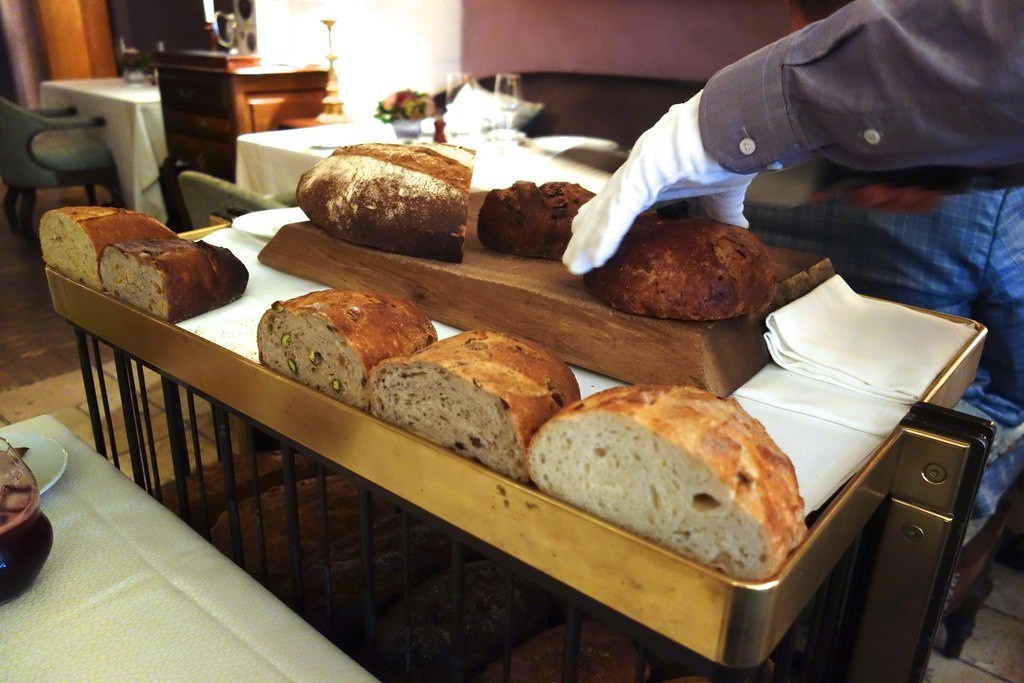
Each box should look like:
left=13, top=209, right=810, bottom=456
left=562, top=88, right=749, bottom=274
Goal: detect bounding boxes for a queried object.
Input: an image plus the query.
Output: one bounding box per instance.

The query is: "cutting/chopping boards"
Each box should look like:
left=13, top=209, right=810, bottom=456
left=258, top=191, right=833, bottom=396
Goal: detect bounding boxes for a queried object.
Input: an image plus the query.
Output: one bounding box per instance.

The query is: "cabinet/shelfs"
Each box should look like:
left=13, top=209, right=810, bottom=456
left=150, top=54, right=328, bottom=228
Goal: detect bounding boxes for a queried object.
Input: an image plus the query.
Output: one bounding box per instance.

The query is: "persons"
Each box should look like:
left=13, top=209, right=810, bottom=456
left=561, top=0, right=1024, bottom=275
left=655, top=187, right=1024, bottom=549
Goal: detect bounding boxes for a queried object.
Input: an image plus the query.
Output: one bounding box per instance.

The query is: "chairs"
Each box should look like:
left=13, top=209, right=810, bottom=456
left=175, top=167, right=291, bottom=226
left=0, top=95, right=125, bottom=248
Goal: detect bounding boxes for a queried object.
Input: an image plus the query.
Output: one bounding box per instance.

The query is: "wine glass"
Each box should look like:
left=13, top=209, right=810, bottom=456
left=493, top=73, right=518, bottom=150
left=446, top=72, right=482, bottom=142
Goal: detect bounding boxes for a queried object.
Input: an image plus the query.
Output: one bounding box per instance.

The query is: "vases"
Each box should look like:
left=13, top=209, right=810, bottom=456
left=391, top=119, right=424, bottom=138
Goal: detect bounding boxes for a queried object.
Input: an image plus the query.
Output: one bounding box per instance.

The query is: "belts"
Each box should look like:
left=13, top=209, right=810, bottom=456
left=821, top=158, right=1024, bottom=195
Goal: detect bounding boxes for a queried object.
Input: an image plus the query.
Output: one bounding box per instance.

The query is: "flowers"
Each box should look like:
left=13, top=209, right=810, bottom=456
left=373, top=88, right=436, bottom=122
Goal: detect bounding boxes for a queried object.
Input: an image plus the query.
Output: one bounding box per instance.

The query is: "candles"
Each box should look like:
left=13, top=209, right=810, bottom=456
left=203, top=0, right=215, bottom=23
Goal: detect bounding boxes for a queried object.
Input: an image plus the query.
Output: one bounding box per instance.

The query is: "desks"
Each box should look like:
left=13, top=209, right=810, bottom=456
left=42, top=77, right=162, bottom=238
left=0, top=414, right=381, bottom=683
left=236, top=113, right=695, bottom=201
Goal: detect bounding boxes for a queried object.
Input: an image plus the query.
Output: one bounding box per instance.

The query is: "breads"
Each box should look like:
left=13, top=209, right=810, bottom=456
left=297, top=142, right=477, bottom=263
left=100, top=239, right=249, bottom=325
left=583, top=209, right=776, bottom=321
left=366, top=328, right=581, bottom=486
left=256, top=287, right=439, bottom=416
left=525, top=383, right=807, bottom=583
left=477, top=181, right=598, bottom=260
left=39, top=206, right=180, bottom=295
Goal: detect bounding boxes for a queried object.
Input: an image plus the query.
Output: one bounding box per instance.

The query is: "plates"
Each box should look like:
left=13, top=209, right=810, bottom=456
left=491, top=130, right=527, bottom=138
left=2, top=432, right=68, bottom=496
